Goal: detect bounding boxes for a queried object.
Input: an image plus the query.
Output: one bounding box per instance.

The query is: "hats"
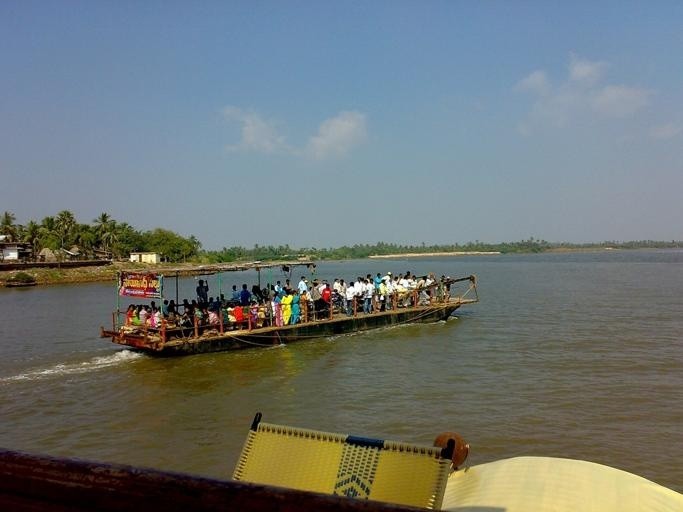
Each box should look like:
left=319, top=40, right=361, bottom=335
left=387, top=271, right=392, bottom=275
left=311, top=279, right=321, bottom=284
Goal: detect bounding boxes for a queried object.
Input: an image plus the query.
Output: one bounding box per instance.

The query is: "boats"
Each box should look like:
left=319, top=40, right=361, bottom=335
left=98, top=257, right=480, bottom=355
left=4, top=279, right=38, bottom=287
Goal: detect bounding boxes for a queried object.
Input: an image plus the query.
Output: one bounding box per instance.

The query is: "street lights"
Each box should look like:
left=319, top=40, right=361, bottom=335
left=50, top=230, right=64, bottom=262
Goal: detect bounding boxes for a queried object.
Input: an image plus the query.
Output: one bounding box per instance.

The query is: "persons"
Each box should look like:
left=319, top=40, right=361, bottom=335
left=122, top=269, right=452, bottom=344
